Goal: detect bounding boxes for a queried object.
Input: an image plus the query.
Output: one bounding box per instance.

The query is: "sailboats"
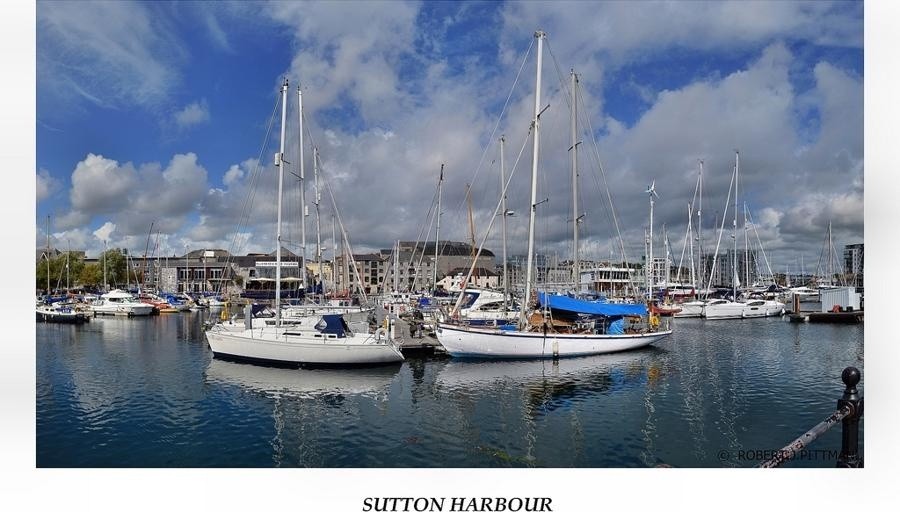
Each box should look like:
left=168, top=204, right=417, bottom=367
left=423, top=345, right=664, bottom=467
left=189, top=354, right=403, bottom=467
